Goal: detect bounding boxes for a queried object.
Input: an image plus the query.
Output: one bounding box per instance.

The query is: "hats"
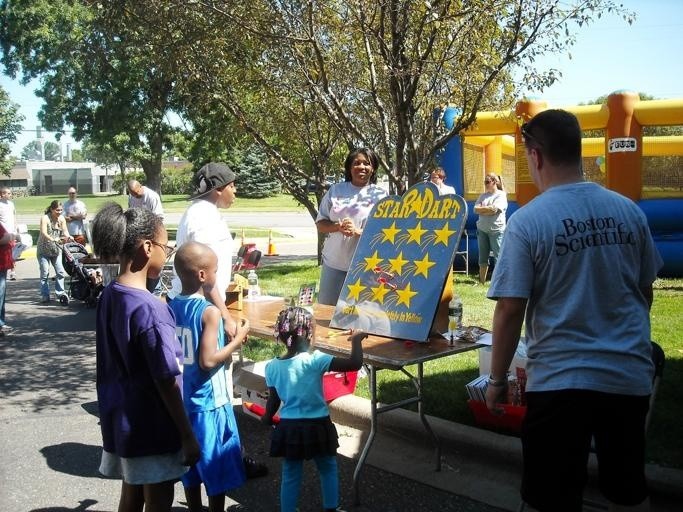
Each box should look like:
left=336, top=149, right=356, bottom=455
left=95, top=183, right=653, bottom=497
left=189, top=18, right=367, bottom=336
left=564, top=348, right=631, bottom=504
left=186, top=162, right=238, bottom=202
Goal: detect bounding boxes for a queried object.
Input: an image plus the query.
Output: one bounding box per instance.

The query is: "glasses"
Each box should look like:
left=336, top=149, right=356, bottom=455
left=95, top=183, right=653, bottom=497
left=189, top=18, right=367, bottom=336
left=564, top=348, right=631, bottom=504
left=485, top=181, right=492, bottom=184
left=520, top=122, right=544, bottom=148
left=148, top=238, right=177, bottom=254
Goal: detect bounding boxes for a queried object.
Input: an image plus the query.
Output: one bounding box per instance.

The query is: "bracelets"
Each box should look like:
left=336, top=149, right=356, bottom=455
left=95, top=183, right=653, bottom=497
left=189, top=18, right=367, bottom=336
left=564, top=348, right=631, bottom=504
left=488, top=375, right=510, bottom=387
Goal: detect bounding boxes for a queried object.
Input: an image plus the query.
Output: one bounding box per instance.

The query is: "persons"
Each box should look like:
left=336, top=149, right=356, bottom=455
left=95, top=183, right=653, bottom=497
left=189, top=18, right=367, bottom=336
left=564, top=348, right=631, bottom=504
left=37, top=200, right=74, bottom=304
left=95, top=201, right=202, bottom=511
left=0, top=187, right=22, bottom=280
left=164, top=161, right=238, bottom=342
left=484, top=109, right=664, bottom=512
left=0, top=221, right=21, bottom=336
left=474, top=173, right=509, bottom=283
left=314, top=148, right=390, bottom=306
left=261, top=306, right=369, bottom=512
left=60, top=186, right=88, bottom=248
left=126, top=180, right=164, bottom=223
left=430, top=167, right=455, bottom=196
left=165, top=241, right=251, bottom=511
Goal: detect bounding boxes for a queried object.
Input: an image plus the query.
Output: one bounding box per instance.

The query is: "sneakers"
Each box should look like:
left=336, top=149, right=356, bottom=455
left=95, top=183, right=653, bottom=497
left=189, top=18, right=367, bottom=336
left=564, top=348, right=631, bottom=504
left=243, top=456, right=268, bottom=478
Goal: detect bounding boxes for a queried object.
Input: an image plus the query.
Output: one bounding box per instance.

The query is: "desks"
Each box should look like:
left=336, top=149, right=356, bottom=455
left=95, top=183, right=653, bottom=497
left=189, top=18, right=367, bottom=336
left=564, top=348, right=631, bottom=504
left=215, top=291, right=493, bottom=492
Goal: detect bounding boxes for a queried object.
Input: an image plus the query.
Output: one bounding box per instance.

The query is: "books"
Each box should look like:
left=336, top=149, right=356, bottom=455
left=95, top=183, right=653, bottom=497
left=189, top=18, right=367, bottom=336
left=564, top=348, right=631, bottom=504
left=465, top=375, right=490, bottom=402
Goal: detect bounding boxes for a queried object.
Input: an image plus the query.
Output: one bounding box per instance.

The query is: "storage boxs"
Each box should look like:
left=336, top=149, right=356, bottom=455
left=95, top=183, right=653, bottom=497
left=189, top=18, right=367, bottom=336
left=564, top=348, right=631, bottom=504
left=319, top=365, right=359, bottom=406
left=238, top=359, right=304, bottom=442
left=461, top=389, right=525, bottom=437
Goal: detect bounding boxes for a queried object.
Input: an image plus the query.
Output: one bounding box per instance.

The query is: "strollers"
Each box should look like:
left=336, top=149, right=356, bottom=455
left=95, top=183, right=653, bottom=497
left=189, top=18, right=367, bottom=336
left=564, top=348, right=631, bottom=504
left=53, top=238, right=100, bottom=309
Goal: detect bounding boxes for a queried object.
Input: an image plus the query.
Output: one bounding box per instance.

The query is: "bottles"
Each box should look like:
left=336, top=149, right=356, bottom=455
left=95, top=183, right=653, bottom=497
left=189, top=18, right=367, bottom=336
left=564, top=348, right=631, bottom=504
left=449, top=294, right=463, bottom=337
left=247, top=267, right=261, bottom=305
